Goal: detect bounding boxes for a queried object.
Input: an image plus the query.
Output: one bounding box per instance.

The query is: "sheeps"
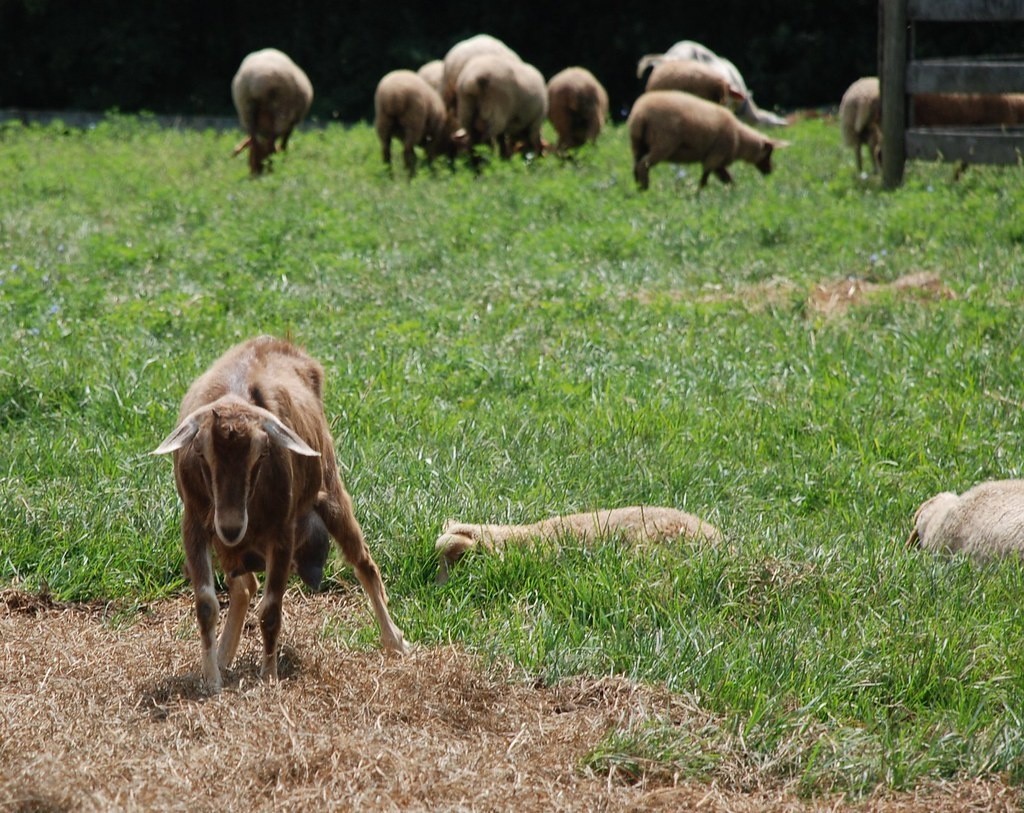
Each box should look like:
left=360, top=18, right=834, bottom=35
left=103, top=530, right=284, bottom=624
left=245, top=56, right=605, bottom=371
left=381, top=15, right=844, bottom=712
left=148, top=336, right=409, bottom=695
left=638, top=40, right=788, bottom=127
left=838, top=76, right=882, bottom=177
left=231, top=49, right=313, bottom=177
left=903, top=479, right=1024, bottom=573
left=547, top=66, right=609, bottom=150
left=628, top=91, right=774, bottom=191
left=435, top=507, right=730, bottom=585
left=374, top=33, right=549, bottom=186
